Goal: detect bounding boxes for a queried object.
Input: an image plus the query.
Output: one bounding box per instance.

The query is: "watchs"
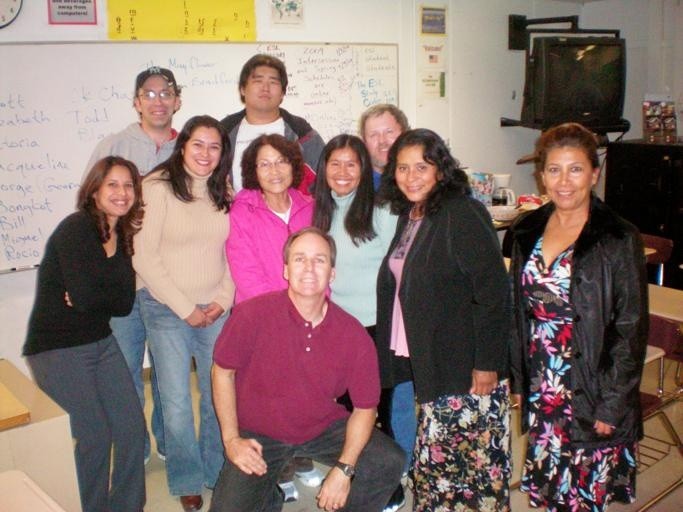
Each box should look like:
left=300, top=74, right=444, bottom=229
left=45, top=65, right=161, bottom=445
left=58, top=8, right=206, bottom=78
left=336, top=462, right=355, bottom=477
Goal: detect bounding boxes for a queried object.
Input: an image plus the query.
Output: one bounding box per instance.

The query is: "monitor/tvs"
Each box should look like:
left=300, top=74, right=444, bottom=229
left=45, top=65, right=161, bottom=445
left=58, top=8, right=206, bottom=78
left=520, top=36, right=626, bottom=123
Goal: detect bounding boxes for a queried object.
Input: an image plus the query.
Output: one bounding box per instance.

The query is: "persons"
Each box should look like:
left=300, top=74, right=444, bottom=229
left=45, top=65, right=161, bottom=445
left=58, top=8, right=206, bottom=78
left=362, top=104, right=411, bottom=192
left=81, top=66, right=188, bottom=466
left=502, top=123, right=649, bottom=512
left=23, top=156, right=146, bottom=512
left=226, top=133, right=325, bottom=502
left=132, top=116, right=236, bottom=512
left=312, top=134, right=407, bottom=512
left=210, top=227, right=407, bottom=512
left=376, top=127, right=511, bottom=512
left=216, top=53, right=327, bottom=202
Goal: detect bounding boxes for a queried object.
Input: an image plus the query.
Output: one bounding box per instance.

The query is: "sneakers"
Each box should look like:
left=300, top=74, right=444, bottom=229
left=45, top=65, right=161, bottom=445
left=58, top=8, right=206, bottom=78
left=278, top=481, right=299, bottom=502
left=294, top=467, right=324, bottom=488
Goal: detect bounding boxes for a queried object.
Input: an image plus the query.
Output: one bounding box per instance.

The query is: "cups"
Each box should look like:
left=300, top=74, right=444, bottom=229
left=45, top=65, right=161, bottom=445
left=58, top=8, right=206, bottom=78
left=462, top=173, right=516, bottom=206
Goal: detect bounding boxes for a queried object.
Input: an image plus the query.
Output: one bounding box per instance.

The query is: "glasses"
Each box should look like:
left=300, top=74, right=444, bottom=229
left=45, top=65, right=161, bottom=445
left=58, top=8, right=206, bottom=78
left=139, top=90, right=175, bottom=100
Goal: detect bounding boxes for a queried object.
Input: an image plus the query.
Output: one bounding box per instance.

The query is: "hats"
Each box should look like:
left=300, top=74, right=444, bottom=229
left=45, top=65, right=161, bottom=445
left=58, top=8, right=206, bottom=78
left=135, top=66, right=176, bottom=97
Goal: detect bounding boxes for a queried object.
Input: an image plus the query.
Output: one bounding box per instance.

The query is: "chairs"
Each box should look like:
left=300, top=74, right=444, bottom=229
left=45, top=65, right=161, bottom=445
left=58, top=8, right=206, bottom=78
left=640, top=230, right=673, bottom=285
left=643, top=345, right=666, bottom=397
left=635, top=391, right=683, bottom=512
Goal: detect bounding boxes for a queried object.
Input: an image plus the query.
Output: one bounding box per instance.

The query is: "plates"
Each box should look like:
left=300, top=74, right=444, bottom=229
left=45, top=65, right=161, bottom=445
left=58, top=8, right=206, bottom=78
left=488, top=205, right=520, bottom=222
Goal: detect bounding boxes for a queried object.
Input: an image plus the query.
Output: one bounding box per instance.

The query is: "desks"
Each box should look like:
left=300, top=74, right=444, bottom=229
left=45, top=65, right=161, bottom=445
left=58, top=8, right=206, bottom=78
left=0, top=357, right=82, bottom=512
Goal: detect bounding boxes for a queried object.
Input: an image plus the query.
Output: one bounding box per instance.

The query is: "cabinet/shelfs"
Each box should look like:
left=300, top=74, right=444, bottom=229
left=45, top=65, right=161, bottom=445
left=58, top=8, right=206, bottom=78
left=604, top=139, right=683, bottom=290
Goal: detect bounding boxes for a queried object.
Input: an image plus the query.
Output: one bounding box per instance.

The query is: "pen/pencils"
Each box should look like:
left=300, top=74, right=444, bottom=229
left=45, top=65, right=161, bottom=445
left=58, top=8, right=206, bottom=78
left=9, top=265, right=42, bottom=271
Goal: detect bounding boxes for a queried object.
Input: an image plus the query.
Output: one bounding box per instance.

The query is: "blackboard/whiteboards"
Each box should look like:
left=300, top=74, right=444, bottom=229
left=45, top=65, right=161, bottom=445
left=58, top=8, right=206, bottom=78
left=0, top=43, right=400, bottom=278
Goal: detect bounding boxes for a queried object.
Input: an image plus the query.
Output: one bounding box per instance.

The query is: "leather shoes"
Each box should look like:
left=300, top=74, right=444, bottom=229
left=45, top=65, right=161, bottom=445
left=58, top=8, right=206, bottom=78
left=180, top=495, right=203, bottom=512
